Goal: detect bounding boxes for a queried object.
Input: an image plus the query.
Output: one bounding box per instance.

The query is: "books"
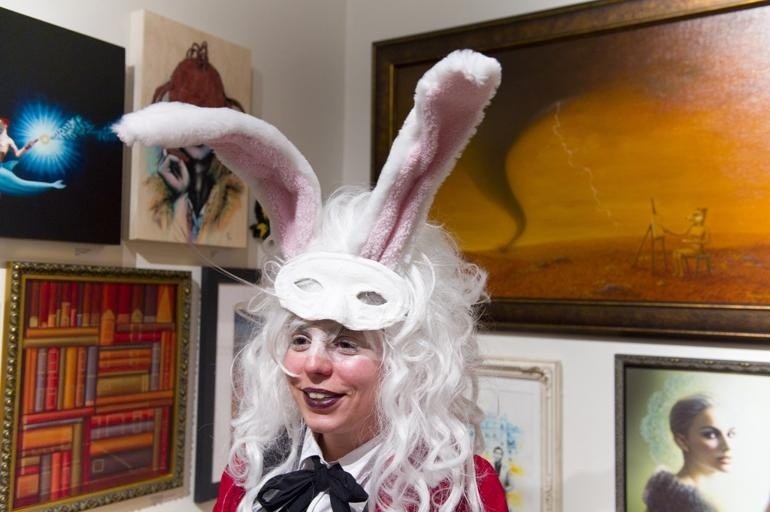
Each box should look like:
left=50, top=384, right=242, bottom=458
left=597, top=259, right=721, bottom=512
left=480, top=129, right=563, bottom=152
left=11, top=277, right=180, bottom=511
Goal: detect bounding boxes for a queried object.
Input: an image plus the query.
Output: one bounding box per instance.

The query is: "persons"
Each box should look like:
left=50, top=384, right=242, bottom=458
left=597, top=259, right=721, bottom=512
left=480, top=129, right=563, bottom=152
left=108, top=43, right=510, bottom=512
left=641, top=393, right=770, bottom=512
left=0, top=117, right=40, bottom=165
left=489, top=446, right=513, bottom=492
left=138, top=44, right=250, bottom=245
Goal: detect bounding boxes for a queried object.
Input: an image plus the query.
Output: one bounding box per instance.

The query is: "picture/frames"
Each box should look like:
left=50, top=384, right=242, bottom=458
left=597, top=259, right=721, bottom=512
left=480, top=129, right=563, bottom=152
left=615, top=354, right=770, bottom=511
left=461, top=354, right=564, bottom=512
left=369, top=1, right=770, bottom=344
left=0, top=263, right=190, bottom=508
left=195, top=263, right=263, bottom=500
